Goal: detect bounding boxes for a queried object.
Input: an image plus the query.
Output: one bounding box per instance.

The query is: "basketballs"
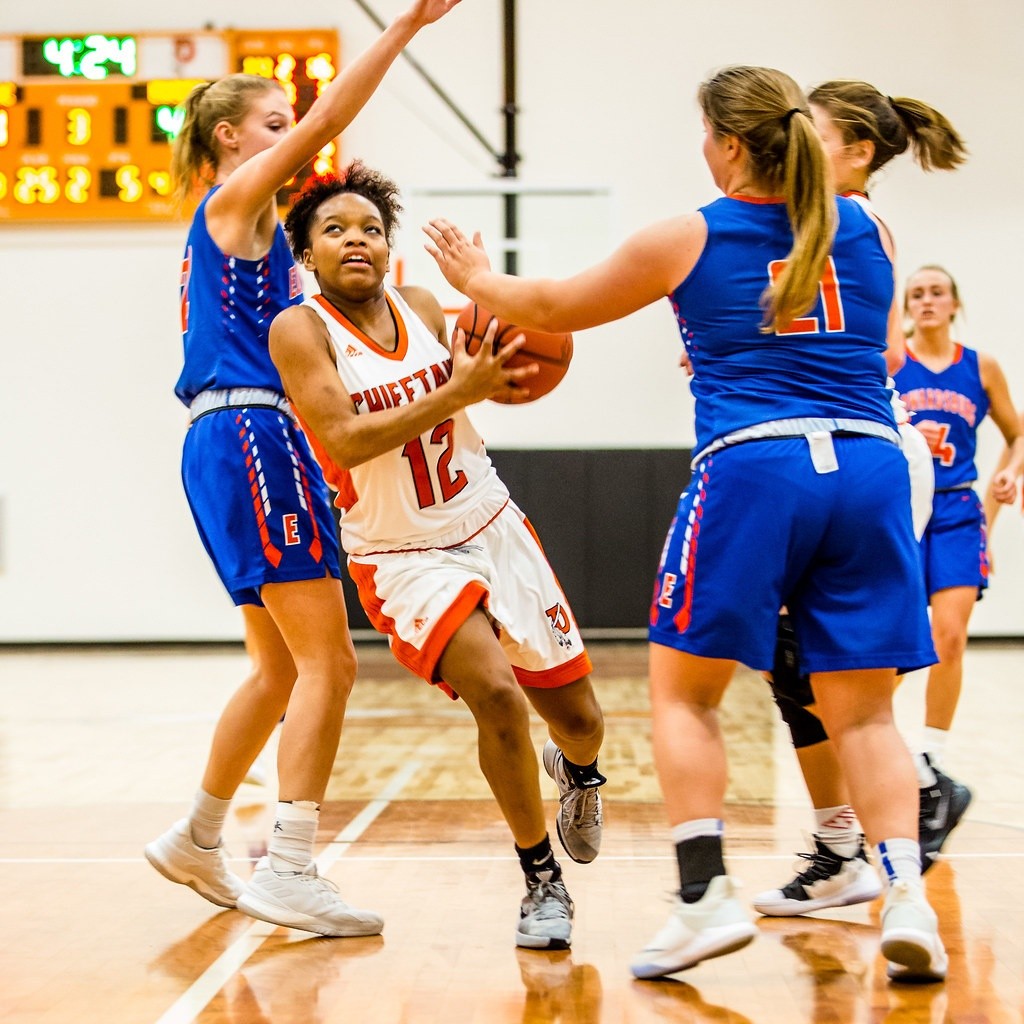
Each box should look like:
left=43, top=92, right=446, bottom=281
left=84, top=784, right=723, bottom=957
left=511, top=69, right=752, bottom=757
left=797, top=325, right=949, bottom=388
left=450, top=299, right=575, bottom=404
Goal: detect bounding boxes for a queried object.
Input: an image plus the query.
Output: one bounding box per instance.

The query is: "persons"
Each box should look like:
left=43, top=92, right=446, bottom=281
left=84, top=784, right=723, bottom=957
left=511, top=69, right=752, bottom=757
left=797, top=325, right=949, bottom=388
left=981, top=406, right=1024, bottom=574
left=142, top=0, right=470, bottom=937
left=422, top=68, right=953, bottom=988
left=267, top=155, right=604, bottom=949
left=893, top=264, right=1024, bottom=775
left=753, top=80, right=975, bottom=919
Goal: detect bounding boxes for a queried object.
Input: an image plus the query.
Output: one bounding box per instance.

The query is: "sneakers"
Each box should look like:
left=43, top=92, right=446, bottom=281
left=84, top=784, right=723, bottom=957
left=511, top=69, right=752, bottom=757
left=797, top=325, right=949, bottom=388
left=515, top=866, right=574, bottom=951
left=542, top=737, right=608, bottom=864
left=630, top=877, right=756, bottom=981
left=879, top=877, right=950, bottom=984
left=752, top=840, right=885, bottom=918
left=919, top=749, right=970, bottom=875
left=143, top=817, right=248, bottom=910
left=235, top=855, right=385, bottom=936
